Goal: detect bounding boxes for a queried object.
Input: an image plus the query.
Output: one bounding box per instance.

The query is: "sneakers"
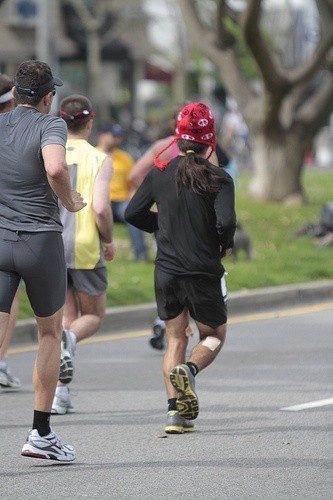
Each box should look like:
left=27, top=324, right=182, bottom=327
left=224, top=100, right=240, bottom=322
left=21, top=427, right=77, bottom=461
left=169, top=364, right=200, bottom=421
left=59, top=329, right=75, bottom=385
left=165, top=409, right=195, bottom=434
left=149, top=318, right=168, bottom=352
left=0, top=364, right=24, bottom=388
left=51, top=387, right=76, bottom=414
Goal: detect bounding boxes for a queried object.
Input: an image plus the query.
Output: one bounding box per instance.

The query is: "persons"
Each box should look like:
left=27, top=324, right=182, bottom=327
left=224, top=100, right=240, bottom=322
left=125, top=102, right=236, bottom=433
left=130, top=108, right=228, bottom=351
left=51, top=95, right=115, bottom=413
left=94, top=122, right=150, bottom=263
left=0, top=59, right=86, bottom=461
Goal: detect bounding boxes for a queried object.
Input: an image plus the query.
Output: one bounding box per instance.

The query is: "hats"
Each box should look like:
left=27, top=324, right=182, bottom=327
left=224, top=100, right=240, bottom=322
left=59, top=107, right=96, bottom=123
left=174, top=101, right=218, bottom=148
left=14, top=78, right=63, bottom=97
